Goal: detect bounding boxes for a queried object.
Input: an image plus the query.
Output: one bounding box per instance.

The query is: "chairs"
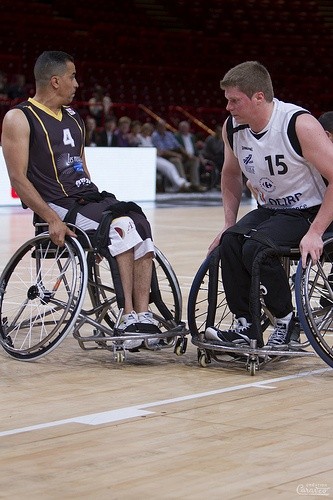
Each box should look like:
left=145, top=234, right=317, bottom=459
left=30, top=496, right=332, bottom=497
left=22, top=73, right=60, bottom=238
left=0, top=0, right=333, bottom=143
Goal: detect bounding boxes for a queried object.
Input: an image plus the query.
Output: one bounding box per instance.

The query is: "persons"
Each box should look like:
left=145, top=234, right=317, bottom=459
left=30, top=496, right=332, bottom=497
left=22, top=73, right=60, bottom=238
left=0, top=74, right=225, bottom=192
left=318, top=110, right=333, bottom=141
left=204, top=62, right=333, bottom=356
left=0, top=52, right=162, bottom=350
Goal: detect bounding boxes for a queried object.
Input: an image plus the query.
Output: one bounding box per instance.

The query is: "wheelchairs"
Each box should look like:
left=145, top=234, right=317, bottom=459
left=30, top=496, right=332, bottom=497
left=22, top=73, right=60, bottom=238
left=186, top=232, right=333, bottom=376
left=0, top=198, right=190, bottom=363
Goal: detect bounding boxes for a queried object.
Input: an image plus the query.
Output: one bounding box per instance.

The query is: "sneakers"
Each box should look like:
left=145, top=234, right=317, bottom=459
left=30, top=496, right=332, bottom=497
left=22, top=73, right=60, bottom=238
left=118, top=311, right=163, bottom=348
left=205, top=317, right=258, bottom=346
left=260, top=314, right=294, bottom=351
